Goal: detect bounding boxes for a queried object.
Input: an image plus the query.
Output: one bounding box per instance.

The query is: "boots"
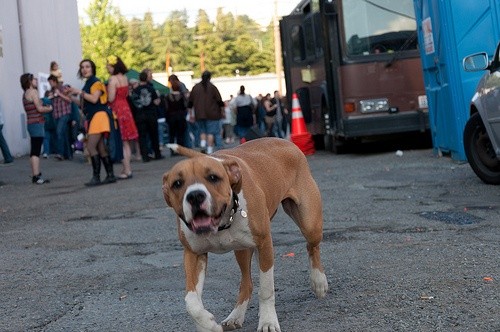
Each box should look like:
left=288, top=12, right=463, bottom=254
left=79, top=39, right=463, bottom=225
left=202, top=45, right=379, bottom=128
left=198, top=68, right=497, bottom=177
left=101, top=154, right=116, bottom=184
left=84, top=154, right=101, bottom=186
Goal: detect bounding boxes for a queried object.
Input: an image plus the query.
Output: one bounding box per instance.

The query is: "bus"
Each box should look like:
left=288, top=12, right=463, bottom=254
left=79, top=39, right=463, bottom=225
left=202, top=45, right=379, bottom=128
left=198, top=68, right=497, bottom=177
left=277, top=0, right=432, bottom=154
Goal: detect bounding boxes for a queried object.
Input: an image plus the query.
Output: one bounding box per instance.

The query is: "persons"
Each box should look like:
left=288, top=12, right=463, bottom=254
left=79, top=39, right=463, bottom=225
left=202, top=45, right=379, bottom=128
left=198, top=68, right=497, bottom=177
left=180, top=89, right=270, bottom=150
left=234, top=85, right=254, bottom=143
left=160, top=82, right=188, bottom=156
left=141, top=68, right=171, bottom=158
left=168, top=74, right=193, bottom=150
left=56, top=76, right=156, bottom=163
left=187, top=70, right=227, bottom=155
left=64, top=59, right=116, bottom=186
left=127, top=72, right=165, bottom=162
left=20, top=74, right=53, bottom=184
left=47, top=61, right=63, bottom=99
left=40, top=90, right=54, bottom=159
left=270, top=91, right=284, bottom=138
left=263, top=93, right=278, bottom=137
left=47, top=75, right=73, bottom=160
left=105, top=54, right=139, bottom=179
left=280, top=96, right=290, bottom=138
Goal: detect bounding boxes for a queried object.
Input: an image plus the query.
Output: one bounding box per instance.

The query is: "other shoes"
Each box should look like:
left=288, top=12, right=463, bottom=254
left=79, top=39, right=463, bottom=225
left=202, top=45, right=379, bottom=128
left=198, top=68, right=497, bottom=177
left=153, top=154, right=165, bottom=160
left=32, top=176, right=50, bottom=184
left=144, top=156, right=154, bottom=162
left=117, top=174, right=132, bottom=180
left=43, top=153, right=48, bottom=159
left=55, top=155, right=64, bottom=160
left=200, top=146, right=206, bottom=153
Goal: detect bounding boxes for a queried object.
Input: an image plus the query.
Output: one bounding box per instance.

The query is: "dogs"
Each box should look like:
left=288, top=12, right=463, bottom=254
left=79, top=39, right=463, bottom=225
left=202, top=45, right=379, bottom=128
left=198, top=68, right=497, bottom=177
left=161, top=137, right=328, bottom=329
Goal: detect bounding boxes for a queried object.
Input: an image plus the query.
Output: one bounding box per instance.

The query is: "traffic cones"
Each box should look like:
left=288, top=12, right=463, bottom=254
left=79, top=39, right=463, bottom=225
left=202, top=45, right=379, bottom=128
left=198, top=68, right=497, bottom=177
left=290, top=92, right=315, bottom=155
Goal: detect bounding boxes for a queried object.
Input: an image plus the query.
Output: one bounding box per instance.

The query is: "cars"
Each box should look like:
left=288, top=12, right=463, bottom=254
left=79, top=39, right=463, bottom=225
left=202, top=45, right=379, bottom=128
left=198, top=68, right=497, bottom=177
left=463, top=39, right=500, bottom=185
